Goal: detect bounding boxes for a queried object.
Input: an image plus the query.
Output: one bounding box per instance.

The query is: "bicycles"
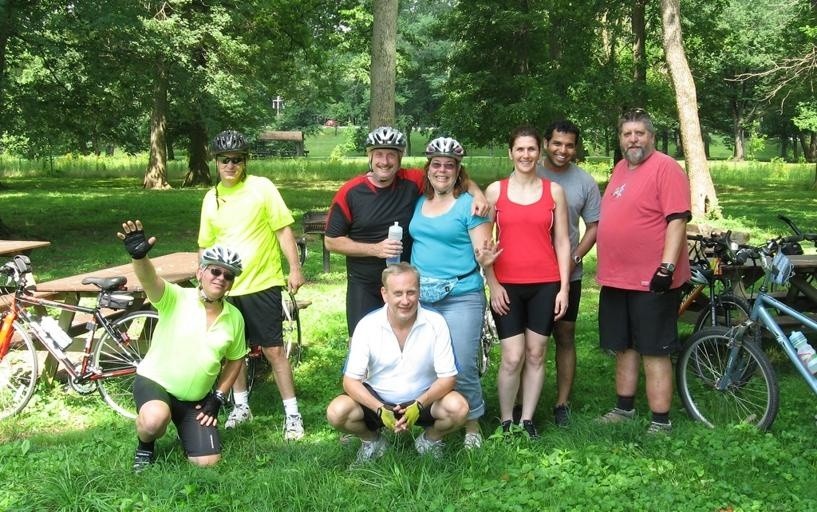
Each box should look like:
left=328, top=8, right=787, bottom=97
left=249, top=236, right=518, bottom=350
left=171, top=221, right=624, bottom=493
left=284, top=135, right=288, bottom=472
left=678, top=227, right=765, bottom=383
left=1, top=251, right=161, bottom=426
left=675, top=212, right=817, bottom=436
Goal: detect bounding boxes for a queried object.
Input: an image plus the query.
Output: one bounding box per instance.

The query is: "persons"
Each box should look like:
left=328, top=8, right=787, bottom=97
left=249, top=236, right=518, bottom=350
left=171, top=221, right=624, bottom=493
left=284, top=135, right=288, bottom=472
left=325, top=261, right=471, bottom=463
left=322, top=124, right=492, bottom=386
left=486, top=127, right=572, bottom=436
left=408, top=136, right=505, bottom=453
left=114, top=218, right=247, bottom=476
left=592, top=108, right=694, bottom=436
left=197, top=128, right=307, bottom=441
left=512, top=119, right=603, bottom=428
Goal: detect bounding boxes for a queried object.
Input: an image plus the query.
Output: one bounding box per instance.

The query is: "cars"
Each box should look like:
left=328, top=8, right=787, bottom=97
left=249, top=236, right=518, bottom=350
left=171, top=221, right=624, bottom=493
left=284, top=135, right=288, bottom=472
left=324, top=119, right=337, bottom=127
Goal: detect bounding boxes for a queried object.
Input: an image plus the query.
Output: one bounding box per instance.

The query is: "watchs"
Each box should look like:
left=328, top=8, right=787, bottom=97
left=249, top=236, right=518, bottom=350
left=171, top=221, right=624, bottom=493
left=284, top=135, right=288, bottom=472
left=573, top=254, right=582, bottom=265
left=660, top=262, right=677, bottom=272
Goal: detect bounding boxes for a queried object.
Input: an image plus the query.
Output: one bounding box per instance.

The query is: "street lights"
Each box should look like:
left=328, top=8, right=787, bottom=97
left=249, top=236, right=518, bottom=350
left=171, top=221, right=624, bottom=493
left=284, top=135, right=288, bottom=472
left=272, top=95, right=285, bottom=116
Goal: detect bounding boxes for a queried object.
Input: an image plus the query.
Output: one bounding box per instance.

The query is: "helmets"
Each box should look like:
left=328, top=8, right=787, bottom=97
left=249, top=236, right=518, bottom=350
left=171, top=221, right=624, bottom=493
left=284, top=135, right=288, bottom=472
left=685, top=258, right=715, bottom=286
left=212, top=130, right=249, bottom=155
left=198, top=245, right=244, bottom=277
left=424, top=136, right=465, bottom=162
left=366, top=126, right=407, bottom=152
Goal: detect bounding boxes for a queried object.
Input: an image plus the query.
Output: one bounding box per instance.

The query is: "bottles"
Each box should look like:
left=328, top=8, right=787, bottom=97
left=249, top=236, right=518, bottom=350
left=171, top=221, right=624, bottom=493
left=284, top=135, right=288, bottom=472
left=788, top=329, right=816, bottom=375
left=384, top=221, right=404, bottom=267
left=41, top=314, right=73, bottom=351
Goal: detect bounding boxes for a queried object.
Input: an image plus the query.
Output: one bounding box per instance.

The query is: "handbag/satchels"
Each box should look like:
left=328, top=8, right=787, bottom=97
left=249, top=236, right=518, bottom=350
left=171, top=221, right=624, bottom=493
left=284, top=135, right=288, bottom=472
left=418, top=275, right=459, bottom=304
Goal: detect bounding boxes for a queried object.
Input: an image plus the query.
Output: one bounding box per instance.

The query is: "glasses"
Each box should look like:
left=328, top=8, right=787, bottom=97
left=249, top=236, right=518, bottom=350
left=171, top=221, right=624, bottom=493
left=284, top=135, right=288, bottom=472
left=216, top=154, right=245, bottom=165
left=206, top=267, right=235, bottom=281
left=617, top=108, right=648, bottom=116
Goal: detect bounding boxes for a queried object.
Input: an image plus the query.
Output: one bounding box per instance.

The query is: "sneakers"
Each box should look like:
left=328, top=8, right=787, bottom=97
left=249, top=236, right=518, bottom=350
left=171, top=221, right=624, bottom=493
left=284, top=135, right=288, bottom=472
left=499, top=404, right=538, bottom=444
left=224, top=402, right=253, bottom=431
left=414, top=430, right=444, bottom=463
left=133, top=444, right=155, bottom=472
left=463, top=433, right=484, bottom=450
left=350, top=433, right=390, bottom=471
left=646, top=419, right=673, bottom=436
left=553, top=402, right=569, bottom=428
left=592, top=406, right=636, bottom=426
left=284, top=412, right=305, bottom=441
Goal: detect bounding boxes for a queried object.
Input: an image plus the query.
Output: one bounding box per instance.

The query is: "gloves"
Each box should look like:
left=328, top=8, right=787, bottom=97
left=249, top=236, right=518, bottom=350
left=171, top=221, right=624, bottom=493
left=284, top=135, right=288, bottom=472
left=376, top=404, right=403, bottom=433
left=398, top=399, right=425, bottom=430
left=649, top=266, right=674, bottom=292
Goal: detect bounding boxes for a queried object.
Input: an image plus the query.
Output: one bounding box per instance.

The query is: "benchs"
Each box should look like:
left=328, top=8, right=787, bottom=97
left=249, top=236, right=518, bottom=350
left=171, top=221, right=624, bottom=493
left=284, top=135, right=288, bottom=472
left=724, top=287, right=810, bottom=309
left=742, top=310, right=816, bottom=336
left=0, top=287, right=61, bottom=313
left=279, top=297, right=312, bottom=321
left=67, top=295, right=149, bottom=338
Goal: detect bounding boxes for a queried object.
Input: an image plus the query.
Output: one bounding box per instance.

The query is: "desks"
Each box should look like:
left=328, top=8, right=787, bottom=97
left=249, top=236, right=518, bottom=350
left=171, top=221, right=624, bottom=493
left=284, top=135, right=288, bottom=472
left=676, top=250, right=816, bottom=361
left=0, top=238, right=59, bottom=346
left=30, top=250, right=199, bottom=389
